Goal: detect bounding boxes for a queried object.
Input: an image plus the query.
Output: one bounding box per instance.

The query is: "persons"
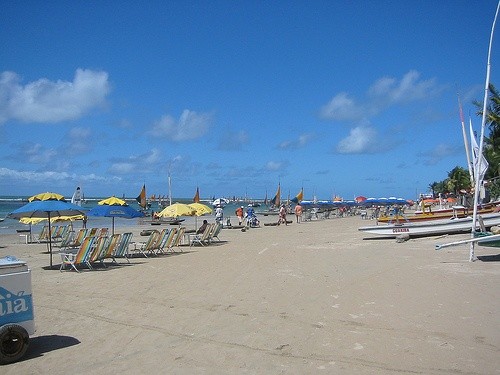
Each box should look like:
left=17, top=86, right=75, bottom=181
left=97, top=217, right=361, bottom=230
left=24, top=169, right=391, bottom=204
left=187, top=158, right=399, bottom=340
left=215, top=205, right=223, bottom=220
left=295, top=203, right=302, bottom=224
left=151, top=210, right=162, bottom=220
left=277, top=205, right=289, bottom=226
left=194, top=220, right=211, bottom=240
left=339, top=204, right=413, bottom=218
left=269, top=201, right=292, bottom=213
left=305, top=208, right=328, bottom=219
left=246, top=204, right=254, bottom=223
left=82, top=215, right=87, bottom=229
left=235, top=206, right=245, bottom=226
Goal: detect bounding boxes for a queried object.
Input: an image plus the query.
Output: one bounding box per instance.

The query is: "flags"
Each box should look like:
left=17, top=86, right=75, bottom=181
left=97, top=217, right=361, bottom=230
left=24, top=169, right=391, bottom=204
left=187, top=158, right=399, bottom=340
left=468, top=115, right=489, bottom=193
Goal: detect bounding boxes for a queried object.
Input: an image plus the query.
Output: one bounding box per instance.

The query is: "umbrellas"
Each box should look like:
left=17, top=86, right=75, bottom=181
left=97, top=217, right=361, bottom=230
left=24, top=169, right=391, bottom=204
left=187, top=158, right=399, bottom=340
left=8, top=191, right=90, bottom=269
left=212, top=197, right=229, bottom=207
left=158, top=202, right=196, bottom=246
left=187, top=202, right=212, bottom=234
left=342, top=197, right=414, bottom=208
left=301, top=200, right=342, bottom=212
left=85, top=195, right=146, bottom=236
left=419, top=197, right=458, bottom=207
left=151, top=194, right=169, bottom=207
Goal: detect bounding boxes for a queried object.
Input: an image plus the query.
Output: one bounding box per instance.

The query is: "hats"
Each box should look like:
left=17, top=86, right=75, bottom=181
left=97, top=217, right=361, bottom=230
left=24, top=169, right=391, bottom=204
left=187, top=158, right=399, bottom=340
left=217, top=205, right=221, bottom=208
left=248, top=204, right=252, bottom=206
left=297, top=203, right=300, bottom=205
left=281, top=204, right=285, bottom=207
left=241, top=206, right=244, bottom=209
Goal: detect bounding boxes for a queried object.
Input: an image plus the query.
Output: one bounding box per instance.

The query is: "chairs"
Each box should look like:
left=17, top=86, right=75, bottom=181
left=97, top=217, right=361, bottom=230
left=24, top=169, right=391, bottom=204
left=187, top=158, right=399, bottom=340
left=17, top=223, right=223, bottom=273
left=322, top=204, right=406, bottom=220
left=452, top=193, right=469, bottom=218
left=421, top=197, right=431, bottom=214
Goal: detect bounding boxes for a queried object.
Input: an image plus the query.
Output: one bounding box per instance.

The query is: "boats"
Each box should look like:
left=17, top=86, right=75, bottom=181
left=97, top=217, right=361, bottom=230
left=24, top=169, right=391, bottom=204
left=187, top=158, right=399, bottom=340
left=357, top=200, right=500, bottom=250
